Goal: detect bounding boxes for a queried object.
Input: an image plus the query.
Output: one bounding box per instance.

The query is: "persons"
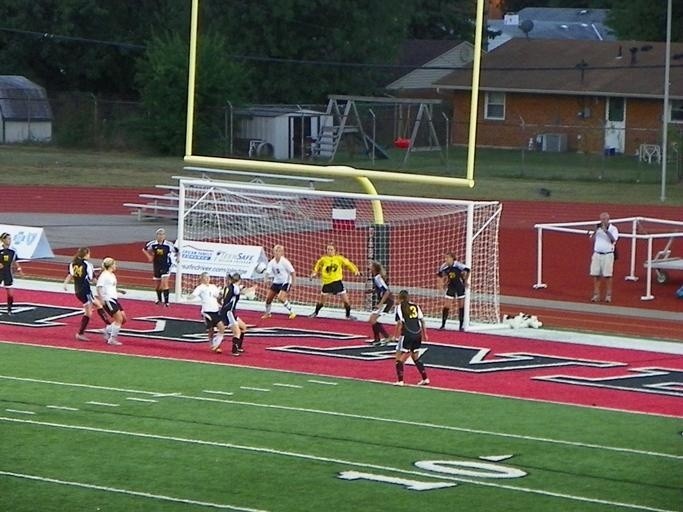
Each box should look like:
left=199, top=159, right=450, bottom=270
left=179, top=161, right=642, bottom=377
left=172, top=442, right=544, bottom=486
left=142, top=227, right=176, bottom=307
left=260, top=244, right=297, bottom=319
left=589, top=212, right=619, bottom=303
left=391, top=290, right=430, bottom=387
left=63, top=247, right=114, bottom=341
left=308, top=244, right=361, bottom=320
left=368, top=260, right=394, bottom=347
left=435, top=252, right=471, bottom=331
left=188, top=272, right=225, bottom=353
left=217, top=272, right=247, bottom=356
left=0, top=233, right=24, bottom=316
left=96, top=257, right=127, bottom=346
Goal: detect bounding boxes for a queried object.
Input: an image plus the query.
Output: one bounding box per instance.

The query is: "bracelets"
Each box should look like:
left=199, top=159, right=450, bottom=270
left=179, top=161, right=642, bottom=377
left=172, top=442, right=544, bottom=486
left=16, top=267, right=22, bottom=271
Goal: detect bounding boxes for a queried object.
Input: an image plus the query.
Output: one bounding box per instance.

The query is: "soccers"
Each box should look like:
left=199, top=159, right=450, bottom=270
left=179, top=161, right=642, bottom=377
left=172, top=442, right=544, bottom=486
left=255, top=262, right=267, bottom=275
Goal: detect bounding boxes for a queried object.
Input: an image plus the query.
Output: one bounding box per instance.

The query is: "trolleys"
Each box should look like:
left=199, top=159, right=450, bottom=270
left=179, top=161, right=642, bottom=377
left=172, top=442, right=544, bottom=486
left=643, top=256, right=681, bottom=283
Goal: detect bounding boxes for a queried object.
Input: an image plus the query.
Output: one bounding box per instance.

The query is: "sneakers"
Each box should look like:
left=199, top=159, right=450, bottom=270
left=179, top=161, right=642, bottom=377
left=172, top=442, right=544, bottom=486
left=439, top=325, right=464, bottom=332
left=75, top=333, right=123, bottom=345
left=155, top=301, right=170, bottom=307
left=392, top=380, right=404, bottom=387
left=370, top=336, right=393, bottom=346
left=591, top=295, right=612, bottom=303
left=260, top=312, right=356, bottom=320
left=210, top=344, right=245, bottom=357
left=417, top=378, right=429, bottom=386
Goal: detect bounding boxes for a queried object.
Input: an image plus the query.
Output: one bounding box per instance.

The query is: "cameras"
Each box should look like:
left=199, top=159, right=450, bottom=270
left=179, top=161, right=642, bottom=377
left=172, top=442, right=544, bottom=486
left=597, top=223, right=602, bottom=227
